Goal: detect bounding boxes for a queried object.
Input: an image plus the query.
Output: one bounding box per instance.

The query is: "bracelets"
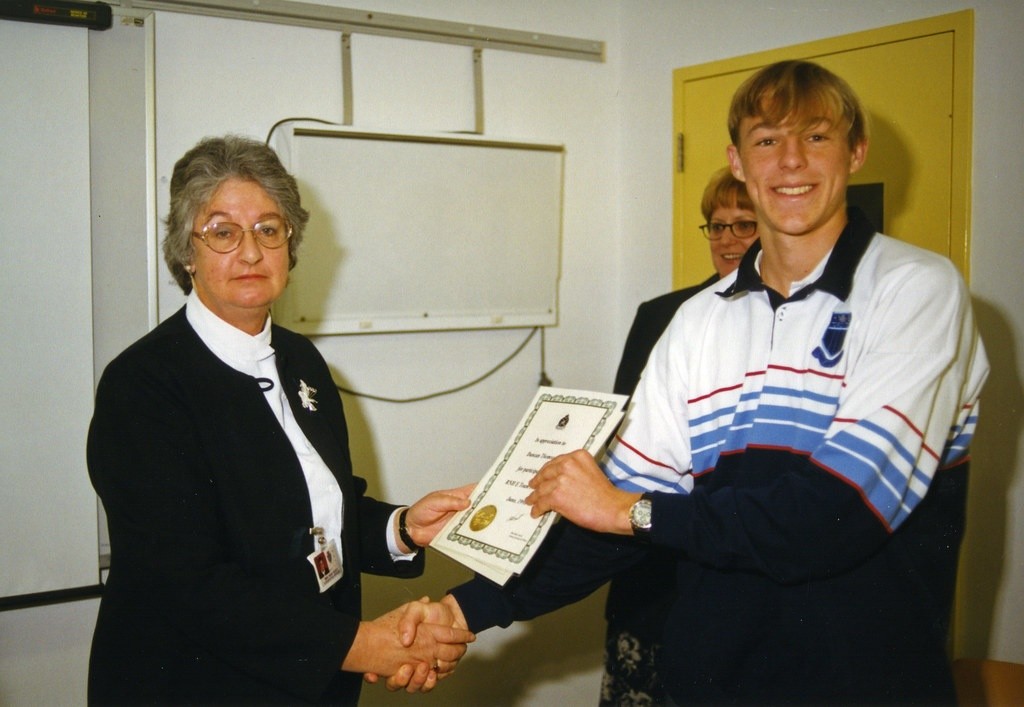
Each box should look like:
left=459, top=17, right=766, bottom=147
left=397, top=508, right=420, bottom=552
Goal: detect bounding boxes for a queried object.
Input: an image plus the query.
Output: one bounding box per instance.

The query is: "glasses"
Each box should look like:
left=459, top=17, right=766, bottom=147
left=699, top=220, right=758, bottom=241
left=189, top=216, right=293, bottom=254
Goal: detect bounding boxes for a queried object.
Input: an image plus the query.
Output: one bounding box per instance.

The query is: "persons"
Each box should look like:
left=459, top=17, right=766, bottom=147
left=365, top=58, right=991, bottom=706
left=608, top=163, right=763, bottom=415
left=81, top=134, right=484, bottom=707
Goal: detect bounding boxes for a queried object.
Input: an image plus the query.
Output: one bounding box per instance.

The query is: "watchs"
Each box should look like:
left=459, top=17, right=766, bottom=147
left=628, top=491, right=656, bottom=544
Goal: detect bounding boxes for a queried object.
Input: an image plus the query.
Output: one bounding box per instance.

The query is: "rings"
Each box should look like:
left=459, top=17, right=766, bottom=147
left=433, top=657, right=438, bottom=671
